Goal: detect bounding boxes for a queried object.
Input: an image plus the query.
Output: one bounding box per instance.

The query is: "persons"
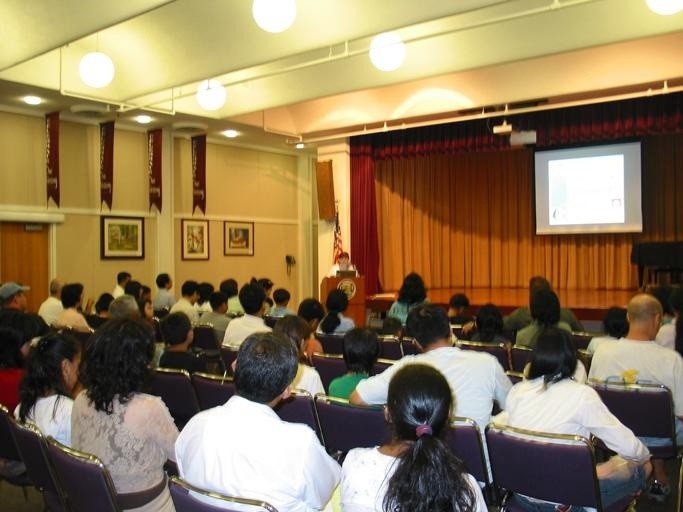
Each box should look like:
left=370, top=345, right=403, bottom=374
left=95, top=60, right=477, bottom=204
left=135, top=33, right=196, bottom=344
left=0, top=273, right=681, bottom=512
left=328, top=251, right=359, bottom=278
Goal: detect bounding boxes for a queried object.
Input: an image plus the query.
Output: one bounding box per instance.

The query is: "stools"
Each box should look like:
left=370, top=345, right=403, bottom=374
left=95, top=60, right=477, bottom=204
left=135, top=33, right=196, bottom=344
left=648, top=267, right=678, bottom=288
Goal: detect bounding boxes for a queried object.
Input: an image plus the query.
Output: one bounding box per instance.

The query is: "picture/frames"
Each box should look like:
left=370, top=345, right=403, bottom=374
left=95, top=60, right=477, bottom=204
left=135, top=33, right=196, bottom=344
left=98, top=214, right=145, bottom=261
left=180, top=218, right=209, bottom=261
left=223, top=220, right=255, bottom=257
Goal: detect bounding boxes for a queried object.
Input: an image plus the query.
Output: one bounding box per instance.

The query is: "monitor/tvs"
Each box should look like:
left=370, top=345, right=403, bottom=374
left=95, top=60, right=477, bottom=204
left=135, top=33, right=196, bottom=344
left=336, top=271, right=356, bottom=277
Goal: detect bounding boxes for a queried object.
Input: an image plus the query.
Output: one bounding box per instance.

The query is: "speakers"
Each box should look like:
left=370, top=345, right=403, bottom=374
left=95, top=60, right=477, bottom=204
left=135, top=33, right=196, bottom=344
left=315, top=160, right=335, bottom=220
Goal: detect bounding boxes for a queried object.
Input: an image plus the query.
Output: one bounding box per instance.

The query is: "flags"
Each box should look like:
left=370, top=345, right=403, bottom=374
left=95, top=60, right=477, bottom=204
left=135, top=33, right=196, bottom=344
left=333, top=211, right=343, bottom=264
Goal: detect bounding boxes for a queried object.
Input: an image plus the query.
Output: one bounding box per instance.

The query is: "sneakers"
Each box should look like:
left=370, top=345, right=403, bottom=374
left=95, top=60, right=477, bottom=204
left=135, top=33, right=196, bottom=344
left=647, top=480, right=671, bottom=503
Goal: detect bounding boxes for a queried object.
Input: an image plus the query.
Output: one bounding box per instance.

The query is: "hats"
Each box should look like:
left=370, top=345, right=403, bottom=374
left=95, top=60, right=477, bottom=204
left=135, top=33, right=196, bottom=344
left=1, top=281, right=31, bottom=298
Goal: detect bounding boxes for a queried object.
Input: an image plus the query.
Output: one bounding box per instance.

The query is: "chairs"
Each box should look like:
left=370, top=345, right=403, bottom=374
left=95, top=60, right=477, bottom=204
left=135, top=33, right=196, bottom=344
left=0, top=304, right=682, bottom=511
